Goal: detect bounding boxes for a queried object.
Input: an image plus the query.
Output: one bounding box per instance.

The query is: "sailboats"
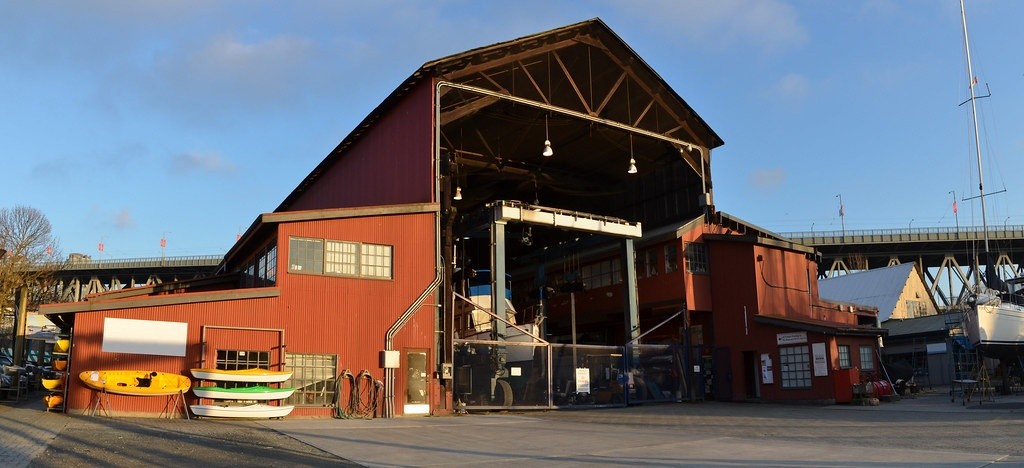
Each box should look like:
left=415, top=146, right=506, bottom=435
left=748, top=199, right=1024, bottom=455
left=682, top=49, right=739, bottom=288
left=958, top=1, right=1024, bottom=358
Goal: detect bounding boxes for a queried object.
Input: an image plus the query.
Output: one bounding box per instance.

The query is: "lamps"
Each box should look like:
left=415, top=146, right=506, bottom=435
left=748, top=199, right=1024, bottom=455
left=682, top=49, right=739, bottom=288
left=542, top=115, right=554, bottom=156
left=627, top=134, right=637, bottom=173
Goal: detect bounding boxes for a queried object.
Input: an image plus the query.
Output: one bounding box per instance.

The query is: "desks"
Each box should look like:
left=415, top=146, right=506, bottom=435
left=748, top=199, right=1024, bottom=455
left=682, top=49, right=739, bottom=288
left=952, top=379, right=982, bottom=406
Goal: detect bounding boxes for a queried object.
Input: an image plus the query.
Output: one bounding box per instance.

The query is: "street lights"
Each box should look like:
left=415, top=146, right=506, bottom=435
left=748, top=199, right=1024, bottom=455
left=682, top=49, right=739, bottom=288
left=949, top=190, right=959, bottom=239
left=836, top=193, right=845, bottom=243
left=909, top=219, right=914, bottom=234
left=1004, top=216, right=1010, bottom=231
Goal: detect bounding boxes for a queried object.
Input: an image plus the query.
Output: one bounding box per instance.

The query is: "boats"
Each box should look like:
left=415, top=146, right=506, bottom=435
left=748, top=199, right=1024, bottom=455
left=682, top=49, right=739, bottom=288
left=45, top=395, right=64, bottom=408
left=52, top=360, right=68, bottom=370
left=454, top=270, right=547, bottom=364
left=190, top=403, right=295, bottom=418
left=42, top=378, right=62, bottom=389
left=192, top=385, right=296, bottom=400
left=190, top=368, right=294, bottom=382
left=79, top=371, right=191, bottom=396
left=54, top=340, right=70, bottom=353
